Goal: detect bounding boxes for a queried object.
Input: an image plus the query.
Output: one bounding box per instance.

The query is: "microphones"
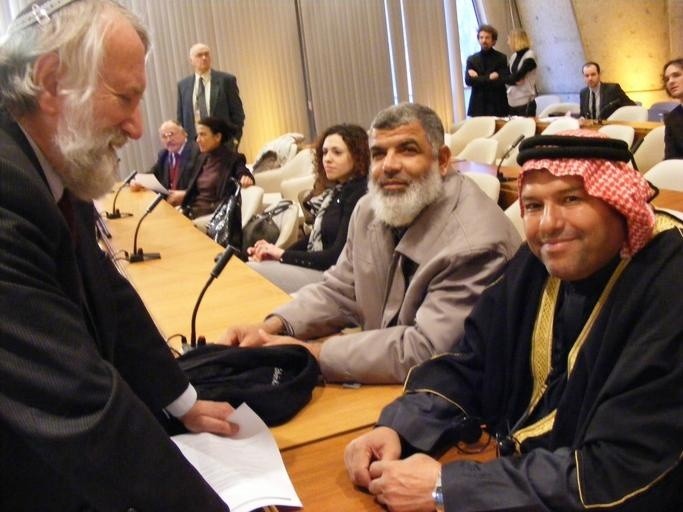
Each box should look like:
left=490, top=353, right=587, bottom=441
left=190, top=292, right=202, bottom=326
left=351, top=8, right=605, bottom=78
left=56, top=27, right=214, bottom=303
left=498, top=134, right=526, bottom=182
left=131, top=192, right=167, bottom=265
left=187, top=247, right=236, bottom=351
left=105, top=170, right=138, bottom=219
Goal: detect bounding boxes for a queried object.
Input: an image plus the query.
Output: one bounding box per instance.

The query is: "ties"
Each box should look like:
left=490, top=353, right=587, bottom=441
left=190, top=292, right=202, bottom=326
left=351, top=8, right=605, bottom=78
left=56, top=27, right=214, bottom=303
left=592, top=91, right=596, bottom=119
left=197, top=77, right=208, bottom=120
left=169, top=153, right=181, bottom=190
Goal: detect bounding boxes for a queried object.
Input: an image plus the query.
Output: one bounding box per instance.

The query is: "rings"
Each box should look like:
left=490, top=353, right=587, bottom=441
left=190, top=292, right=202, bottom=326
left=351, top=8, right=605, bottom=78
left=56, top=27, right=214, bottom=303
left=264, top=246, right=267, bottom=250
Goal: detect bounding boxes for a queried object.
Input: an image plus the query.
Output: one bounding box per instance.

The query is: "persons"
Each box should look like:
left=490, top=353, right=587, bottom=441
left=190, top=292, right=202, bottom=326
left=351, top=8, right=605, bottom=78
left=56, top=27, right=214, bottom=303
left=339, top=127, right=683, bottom=512
left=180, top=115, right=255, bottom=232
left=497, top=27, right=538, bottom=115
left=659, top=58, right=683, bottom=162
left=465, top=24, right=510, bottom=117
left=125, top=118, right=200, bottom=206
left=173, top=42, right=244, bottom=149
left=579, top=62, right=632, bottom=125
left=224, top=102, right=524, bottom=385
left=0, top=0, right=242, bottom=511
left=245, top=122, right=370, bottom=294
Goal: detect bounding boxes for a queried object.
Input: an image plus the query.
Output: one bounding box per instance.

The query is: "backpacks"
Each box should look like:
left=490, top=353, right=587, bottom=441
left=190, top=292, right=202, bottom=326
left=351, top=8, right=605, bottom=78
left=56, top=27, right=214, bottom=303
left=174, top=342, right=320, bottom=429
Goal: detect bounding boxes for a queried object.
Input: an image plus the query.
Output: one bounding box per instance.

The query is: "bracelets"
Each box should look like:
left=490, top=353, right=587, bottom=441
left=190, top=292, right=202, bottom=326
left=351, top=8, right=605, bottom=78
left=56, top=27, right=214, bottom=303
left=430, top=477, right=445, bottom=511
